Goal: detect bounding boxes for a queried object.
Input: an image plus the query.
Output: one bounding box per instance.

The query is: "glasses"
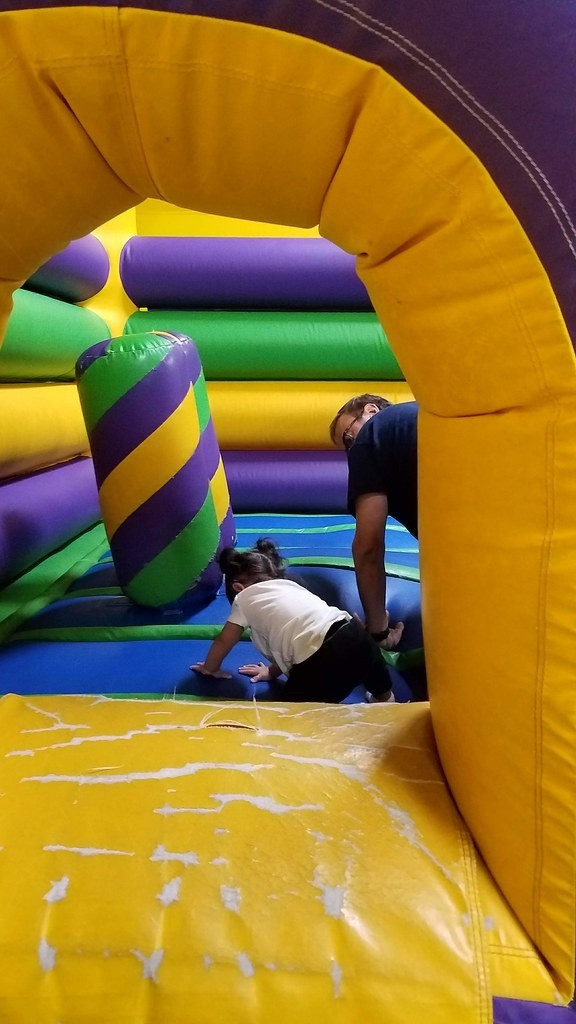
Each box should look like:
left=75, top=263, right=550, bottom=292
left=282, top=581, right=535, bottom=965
left=342, top=410, right=364, bottom=452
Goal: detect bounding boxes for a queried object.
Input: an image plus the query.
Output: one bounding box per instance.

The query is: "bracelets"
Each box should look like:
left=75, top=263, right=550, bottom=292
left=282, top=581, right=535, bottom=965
left=373, top=627, right=391, bottom=642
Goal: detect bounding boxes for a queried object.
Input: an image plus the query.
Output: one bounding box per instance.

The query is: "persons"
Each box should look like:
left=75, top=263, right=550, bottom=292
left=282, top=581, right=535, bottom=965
left=190, top=534, right=396, bottom=704
left=329, top=393, right=418, bottom=650
